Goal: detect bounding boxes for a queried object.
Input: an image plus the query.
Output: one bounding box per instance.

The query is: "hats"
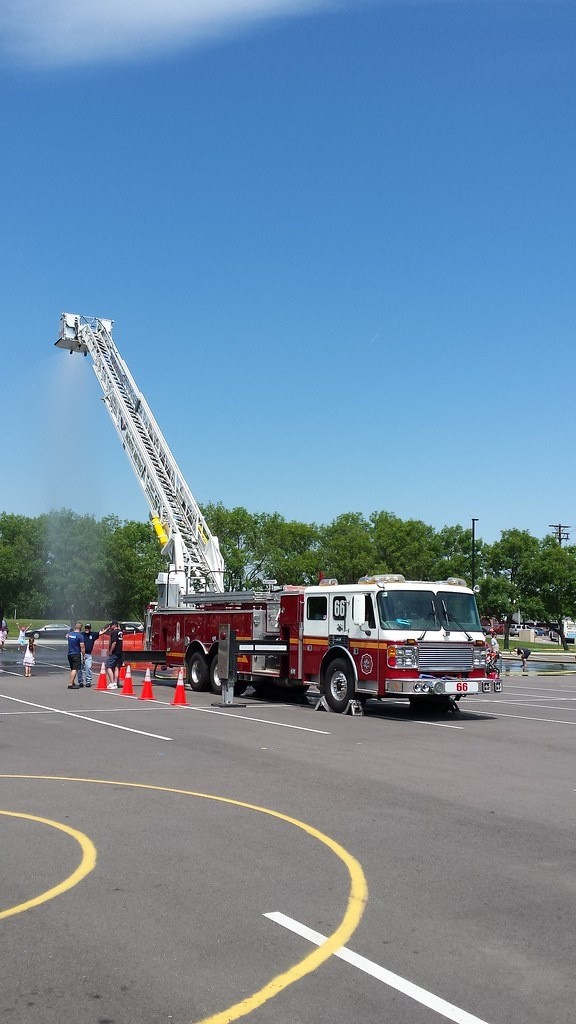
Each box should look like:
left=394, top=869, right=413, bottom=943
left=84, top=623, right=91, bottom=628
left=109, top=621, right=118, bottom=625
left=21, top=627, right=26, bottom=631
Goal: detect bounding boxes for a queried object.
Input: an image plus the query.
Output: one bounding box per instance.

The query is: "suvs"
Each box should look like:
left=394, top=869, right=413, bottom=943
left=509, top=624, right=538, bottom=638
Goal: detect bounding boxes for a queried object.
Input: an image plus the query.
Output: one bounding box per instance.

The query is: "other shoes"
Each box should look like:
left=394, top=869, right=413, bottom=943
left=86, top=683, right=90, bottom=687
left=29, top=674, right=31, bottom=676
left=68, top=685, right=79, bottom=689
left=80, top=682, right=83, bottom=687
left=25, top=674, right=28, bottom=676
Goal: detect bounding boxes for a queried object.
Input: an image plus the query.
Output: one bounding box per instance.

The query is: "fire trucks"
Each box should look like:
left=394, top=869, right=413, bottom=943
left=53, top=310, right=503, bottom=716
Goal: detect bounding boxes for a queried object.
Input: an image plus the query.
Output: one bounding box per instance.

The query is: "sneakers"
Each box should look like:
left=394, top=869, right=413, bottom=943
left=107, top=684, right=115, bottom=689
left=114, top=684, right=118, bottom=689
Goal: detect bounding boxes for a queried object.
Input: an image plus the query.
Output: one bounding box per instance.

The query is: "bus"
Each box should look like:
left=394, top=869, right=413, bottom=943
left=548, top=618, right=576, bottom=642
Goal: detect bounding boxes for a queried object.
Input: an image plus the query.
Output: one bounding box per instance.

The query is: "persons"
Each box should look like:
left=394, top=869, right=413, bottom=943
left=22, top=637, right=38, bottom=678
left=483, top=634, right=491, bottom=658
left=122, top=629, right=128, bottom=637
left=0, top=625, right=11, bottom=653
left=105, top=620, right=125, bottom=691
left=491, top=632, right=501, bottom=658
left=510, top=646, right=531, bottom=667
left=15, top=622, right=33, bottom=653
left=98, top=619, right=114, bottom=636
left=65, top=620, right=87, bottom=692
left=77, top=623, right=111, bottom=688
left=392, top=599, right=423, bottom=624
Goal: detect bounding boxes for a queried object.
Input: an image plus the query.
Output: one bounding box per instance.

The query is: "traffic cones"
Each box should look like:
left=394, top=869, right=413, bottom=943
left=119, top=663, right=136, bottom=695
left=118, top=663, right=126, bottom=680
left=171, top=667, right=190, bottom=705
left=94, top=662, right=109, bottom=690
left=138, top=667, right=156, bottom=700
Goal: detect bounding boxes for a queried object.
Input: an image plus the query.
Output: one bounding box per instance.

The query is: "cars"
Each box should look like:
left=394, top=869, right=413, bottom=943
left=25, top=623, right=71, bottom=640
left=493, top=624, right=503, bottom=635
left=531, top=627, right=546, bottom=636
left=121, top=621, right=145, bottom=633
